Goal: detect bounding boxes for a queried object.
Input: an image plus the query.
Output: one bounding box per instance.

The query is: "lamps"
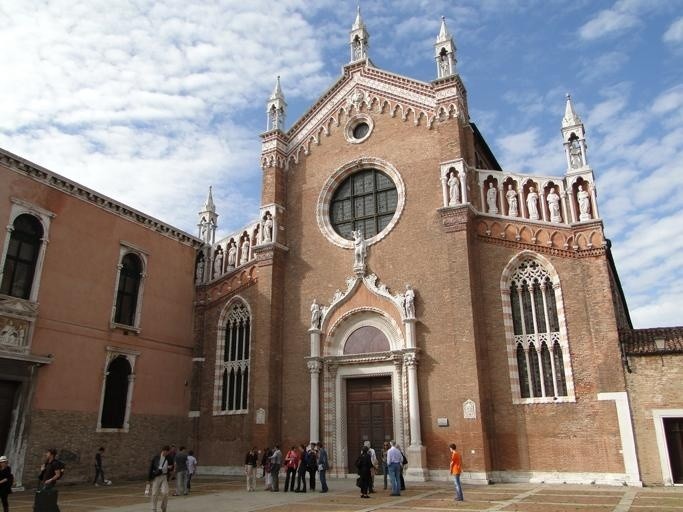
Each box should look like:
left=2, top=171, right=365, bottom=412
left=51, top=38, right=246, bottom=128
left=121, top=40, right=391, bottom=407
left=652, top=332, right=668, bottom=367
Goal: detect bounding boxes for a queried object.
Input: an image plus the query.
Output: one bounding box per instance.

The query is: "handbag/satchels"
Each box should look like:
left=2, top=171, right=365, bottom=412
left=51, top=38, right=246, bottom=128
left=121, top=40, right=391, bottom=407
left=399, top=450, right=407, bottom=464
left=151, top=468, right=162, bottom=476
left=283, top=448, right=291, bottom=465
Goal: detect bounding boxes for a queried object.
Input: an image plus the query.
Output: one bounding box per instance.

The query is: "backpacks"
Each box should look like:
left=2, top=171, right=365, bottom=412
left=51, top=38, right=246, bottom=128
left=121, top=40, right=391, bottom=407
left=44, top=458, right=66, bottom=481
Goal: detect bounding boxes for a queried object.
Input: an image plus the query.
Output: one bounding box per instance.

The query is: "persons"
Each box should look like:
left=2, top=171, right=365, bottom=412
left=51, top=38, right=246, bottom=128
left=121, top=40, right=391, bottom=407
left=448, top=442, right=464, bottom=502
left=310, top=297, right=324, bottom=332
left=0, top=319, right=20, bottom=342
left=254, top=227, right=259, bottom=245
left=185, top=450, right=197, bottom=489
left=16, top=324, right=25, bottom=346
left=351, top=228, right=367, bottom=268
left=172, top=445, right=189, bottom=497
left=523, top=185, right=540, bottom=222
left=446, top=172, right=461, bottom=207
left=575, top=184, right=593, bottom=223
left=165, top=445, right=176, bottom=482
left=194, top=257, right=205, bottom=284
left=92, top=446, right=107, bottom=487
left=261, top=214, right=272, bottom=243
left=238, top=236, right=249, bottom=266
left=485, top=181, right=498, bottom=214
left=504, top=184, right=518, bottom=218
left=362, top=440, right=379, bottom=493
left=353, top=445, right=374, bottom=499
left=32, top=447, right=65, bottom=511
left=225, top=242, right=236, bottom=272
left=146, top=445, right=170, bottom=512
left=403, top=284, right=418, bottom=319
left=0, top=454, right=15, bottom=512
left=241, top=439, right=331, bottom=493
left=395, top=444, right=408, bottom=491
left=377, top=440, right=390, bottom=490
left=385, top=440, right=402, bottom=497
left=211, top=249, right=222, bottom=281
left=545, top=186, right=562, bottom=224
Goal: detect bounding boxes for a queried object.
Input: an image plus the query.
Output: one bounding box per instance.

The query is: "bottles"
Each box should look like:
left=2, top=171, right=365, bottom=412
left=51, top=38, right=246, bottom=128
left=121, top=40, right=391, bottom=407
left=144, top=483, right=150, bottom=496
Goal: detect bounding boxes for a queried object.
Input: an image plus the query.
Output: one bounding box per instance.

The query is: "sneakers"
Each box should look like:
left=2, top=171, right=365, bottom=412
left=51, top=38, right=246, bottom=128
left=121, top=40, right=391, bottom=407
left=246, top=483, right=464, bottom=503
left=172, top=492, right=187, bottom=496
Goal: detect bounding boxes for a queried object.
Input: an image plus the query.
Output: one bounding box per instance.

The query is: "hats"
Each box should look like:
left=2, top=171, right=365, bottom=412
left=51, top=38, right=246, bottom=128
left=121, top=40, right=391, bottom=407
left=0, top=455, right=8, bottom=463
left=44, top=446, right=58, bottom=454
